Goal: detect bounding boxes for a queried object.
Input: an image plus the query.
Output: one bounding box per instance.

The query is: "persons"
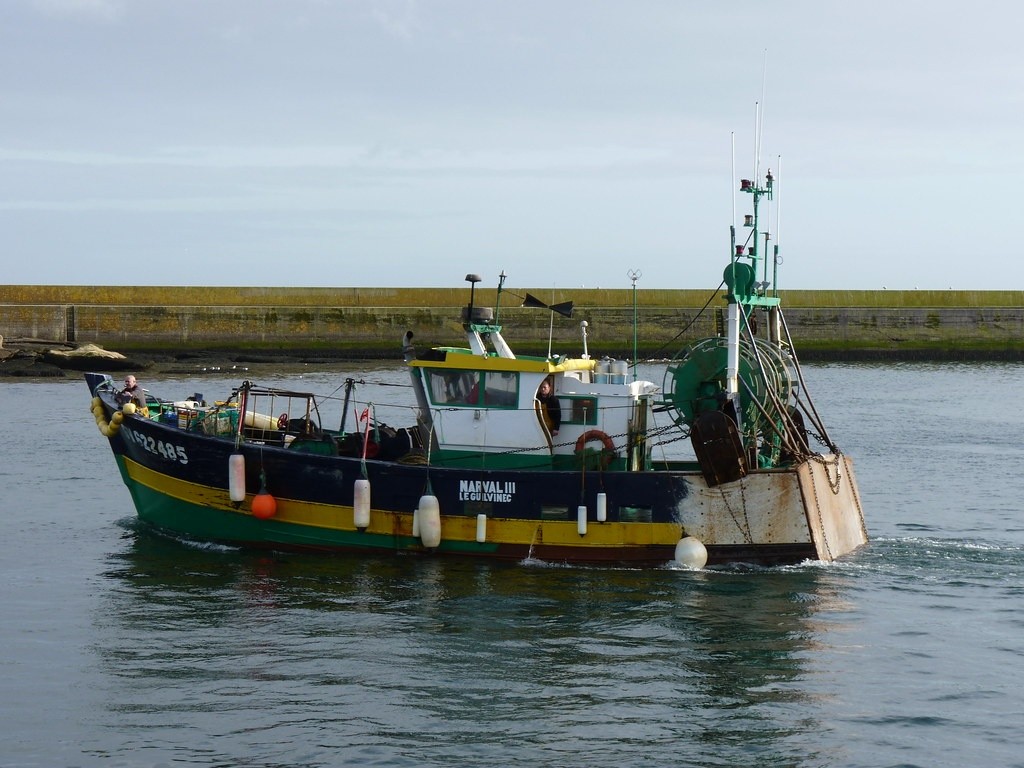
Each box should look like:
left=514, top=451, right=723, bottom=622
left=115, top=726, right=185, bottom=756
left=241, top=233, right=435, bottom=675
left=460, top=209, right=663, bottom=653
left=536, top=381, right=562, bottom=436
left=115, top=374, right=149, bottom=419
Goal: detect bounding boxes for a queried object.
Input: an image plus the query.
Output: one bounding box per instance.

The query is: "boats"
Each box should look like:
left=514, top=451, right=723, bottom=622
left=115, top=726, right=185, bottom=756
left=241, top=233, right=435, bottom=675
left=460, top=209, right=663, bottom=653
left=79, top=100, right=882, bottom=573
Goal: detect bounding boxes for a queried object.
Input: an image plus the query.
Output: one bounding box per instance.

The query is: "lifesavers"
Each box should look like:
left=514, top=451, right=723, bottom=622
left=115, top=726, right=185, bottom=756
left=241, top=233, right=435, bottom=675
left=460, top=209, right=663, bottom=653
left=576, top=429, right=615, bottom=469
